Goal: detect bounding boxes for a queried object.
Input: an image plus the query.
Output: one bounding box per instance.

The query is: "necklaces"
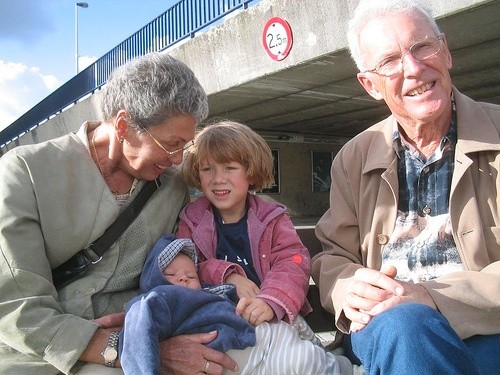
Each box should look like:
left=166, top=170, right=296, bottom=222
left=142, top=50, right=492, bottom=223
left=92, top=128, right=132, bottom=194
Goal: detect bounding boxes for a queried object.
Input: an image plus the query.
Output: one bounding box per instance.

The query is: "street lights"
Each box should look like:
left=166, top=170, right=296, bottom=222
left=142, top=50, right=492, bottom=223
left=75, top=1, right=88, bottom=76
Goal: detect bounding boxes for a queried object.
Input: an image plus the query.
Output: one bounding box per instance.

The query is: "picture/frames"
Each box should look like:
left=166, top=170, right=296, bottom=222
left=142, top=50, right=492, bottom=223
left=311, top=150, right=333, bottom=193
left=255, top=148, right=280, bottom=194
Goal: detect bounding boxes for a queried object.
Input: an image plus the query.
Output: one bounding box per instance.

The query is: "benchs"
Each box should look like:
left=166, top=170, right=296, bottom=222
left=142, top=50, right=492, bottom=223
left=292, top=226, right=336, bottom=332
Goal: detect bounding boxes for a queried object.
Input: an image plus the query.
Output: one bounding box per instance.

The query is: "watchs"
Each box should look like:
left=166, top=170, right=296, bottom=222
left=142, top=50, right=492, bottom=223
left=101, top=330, right=121, bottom=368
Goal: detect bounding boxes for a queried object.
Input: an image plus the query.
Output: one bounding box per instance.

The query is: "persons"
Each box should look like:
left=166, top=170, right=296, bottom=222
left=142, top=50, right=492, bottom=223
left=118, top=232, right=353, bottom=375
left=312, top=1, right=500, bottom=375
left=176, top=120, right=312, bottom=325
left=0, top=52, right=207, bottom=375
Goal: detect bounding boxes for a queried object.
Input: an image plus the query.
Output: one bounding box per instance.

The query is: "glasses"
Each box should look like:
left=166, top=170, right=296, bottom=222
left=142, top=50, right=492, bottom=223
left=361, top=36, right=442, bottom=77
left=137, top=119, right=195, bottom=159
